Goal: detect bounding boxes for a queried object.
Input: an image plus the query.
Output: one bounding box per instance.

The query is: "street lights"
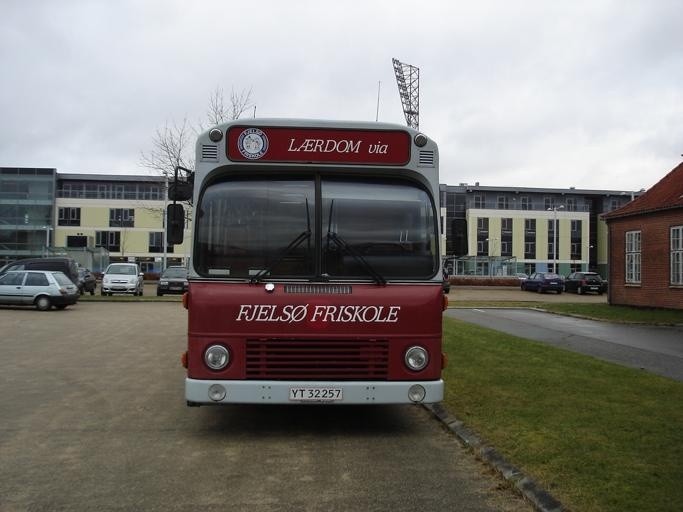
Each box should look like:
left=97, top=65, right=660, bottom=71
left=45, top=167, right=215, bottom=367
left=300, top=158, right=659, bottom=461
left=547, top=205, right=565, bottom=274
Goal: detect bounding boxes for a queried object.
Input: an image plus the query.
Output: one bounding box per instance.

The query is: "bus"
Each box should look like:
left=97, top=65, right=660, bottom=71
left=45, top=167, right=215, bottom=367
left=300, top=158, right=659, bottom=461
left=166, top=117, right=469, bottom=405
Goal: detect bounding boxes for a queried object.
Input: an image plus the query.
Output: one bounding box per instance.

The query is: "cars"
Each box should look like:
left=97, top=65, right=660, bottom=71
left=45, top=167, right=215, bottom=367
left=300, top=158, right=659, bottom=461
left=100, top=262, right=144, bottom=296
left=157, top=266, right=189, bottom=296
left=519, top=272, right=604, bottom=296
left=0, top=256, right=97, bottom=311
